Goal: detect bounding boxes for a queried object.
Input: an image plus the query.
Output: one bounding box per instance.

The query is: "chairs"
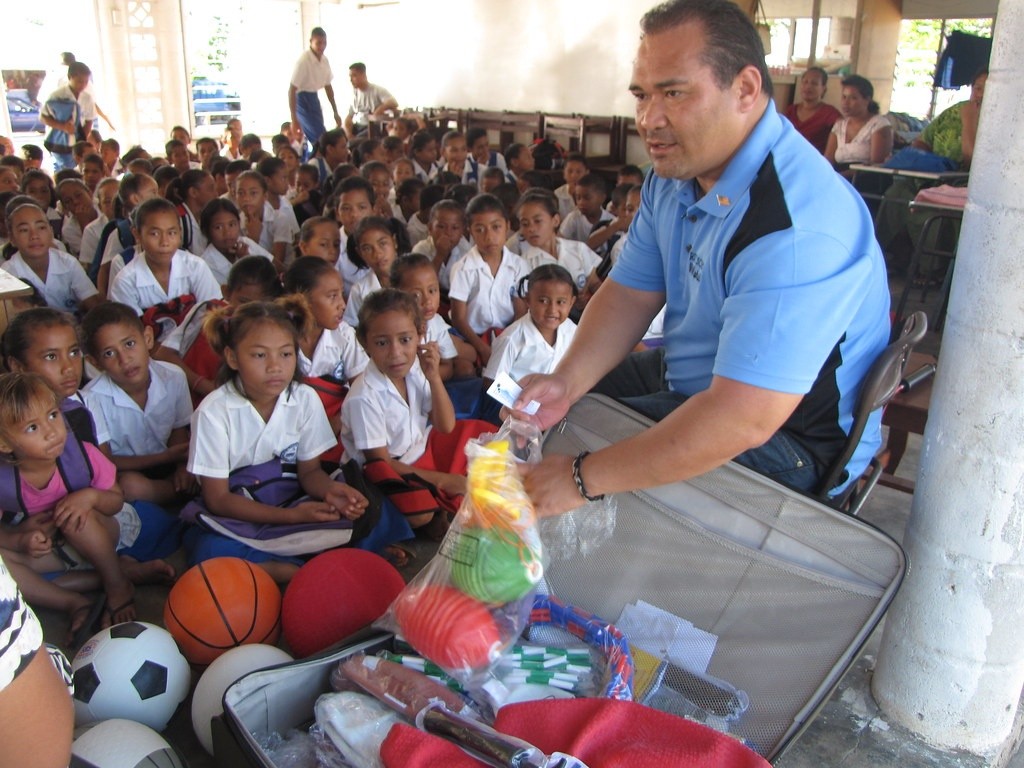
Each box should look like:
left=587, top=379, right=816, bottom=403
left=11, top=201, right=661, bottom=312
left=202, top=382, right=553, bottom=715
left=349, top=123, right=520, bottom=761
left=361, top=106, right=647, bottom=177
left=820, top=310, right=928, bottom=517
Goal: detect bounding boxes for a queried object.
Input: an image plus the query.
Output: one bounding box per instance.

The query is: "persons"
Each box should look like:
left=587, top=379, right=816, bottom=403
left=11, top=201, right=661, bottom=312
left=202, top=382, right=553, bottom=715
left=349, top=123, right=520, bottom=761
left=499, top=0, right=894, bottom=512
left=0, top=114, right=667, bottom=768
left=39, top=52, right=97, bottom=176
left=781, top=67, right=842, bottom=154
left=36, top=52, right=114, bottom=134
left=288, top=27, right=343, bottom=158
left=344, top=63, right=398, bottom=152
left=876, top=67, right=988, bottom=290
left=826, top=76, right=893, bottom=194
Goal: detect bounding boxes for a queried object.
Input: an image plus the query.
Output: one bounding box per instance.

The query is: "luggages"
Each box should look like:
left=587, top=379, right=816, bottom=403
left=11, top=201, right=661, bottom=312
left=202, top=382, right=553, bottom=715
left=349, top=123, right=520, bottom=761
left=210, top=391, right=913, bottom=768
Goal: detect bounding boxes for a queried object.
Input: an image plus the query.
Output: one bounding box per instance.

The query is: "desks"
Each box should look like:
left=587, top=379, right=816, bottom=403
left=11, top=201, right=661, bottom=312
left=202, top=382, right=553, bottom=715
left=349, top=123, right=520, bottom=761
left=844, top=165, right=969, bottom=330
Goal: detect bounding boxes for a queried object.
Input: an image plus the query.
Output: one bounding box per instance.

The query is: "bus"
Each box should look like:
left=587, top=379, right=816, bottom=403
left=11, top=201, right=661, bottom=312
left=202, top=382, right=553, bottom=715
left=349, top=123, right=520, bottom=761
left=192, top=86, right=239, bottom=112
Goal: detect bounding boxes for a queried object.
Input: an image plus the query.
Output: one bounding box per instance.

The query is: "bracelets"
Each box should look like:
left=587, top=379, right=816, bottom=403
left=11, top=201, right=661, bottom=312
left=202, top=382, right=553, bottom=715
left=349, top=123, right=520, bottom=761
left=573, top=450, right=604, bottom=501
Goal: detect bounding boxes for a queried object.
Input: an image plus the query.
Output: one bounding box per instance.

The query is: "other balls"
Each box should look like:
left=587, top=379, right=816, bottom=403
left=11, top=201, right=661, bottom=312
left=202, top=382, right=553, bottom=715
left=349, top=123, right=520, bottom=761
left=192, top=644, right=297, bottom=757
left=69, top=718, right=190, bottom=768
left=282, top=545, right=407, bottom=659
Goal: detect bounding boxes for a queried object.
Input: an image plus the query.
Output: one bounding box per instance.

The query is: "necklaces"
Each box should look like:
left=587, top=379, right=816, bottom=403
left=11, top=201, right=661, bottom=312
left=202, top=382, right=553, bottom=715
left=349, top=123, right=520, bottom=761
left=846, top=134, right=855, bottom=145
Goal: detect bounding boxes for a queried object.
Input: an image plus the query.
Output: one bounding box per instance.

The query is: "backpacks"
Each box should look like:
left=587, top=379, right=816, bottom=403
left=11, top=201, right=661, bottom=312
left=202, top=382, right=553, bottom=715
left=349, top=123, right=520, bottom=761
left=167, top=459, right=382, bottom=557
left=44, top=98, right=86, bottom=154
left=140, top=293, right=197, bottom=341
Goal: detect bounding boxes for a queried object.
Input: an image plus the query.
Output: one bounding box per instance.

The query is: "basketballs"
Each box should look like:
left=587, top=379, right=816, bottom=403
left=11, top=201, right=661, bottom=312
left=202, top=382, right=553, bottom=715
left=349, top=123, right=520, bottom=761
left=164, top=555, right=283, bottom=675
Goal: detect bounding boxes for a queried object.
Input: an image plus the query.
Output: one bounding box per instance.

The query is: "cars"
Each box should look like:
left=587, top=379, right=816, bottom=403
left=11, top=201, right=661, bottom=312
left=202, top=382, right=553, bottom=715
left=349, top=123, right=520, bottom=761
left=7, top=95, right=45, bottom=132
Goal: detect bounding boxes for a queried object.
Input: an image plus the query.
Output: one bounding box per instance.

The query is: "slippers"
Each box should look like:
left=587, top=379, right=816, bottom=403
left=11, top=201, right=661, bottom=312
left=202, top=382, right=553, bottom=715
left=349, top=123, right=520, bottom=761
left=68, top=592, right=106, bottom=645
left=92, top=598, right=136, bottom=635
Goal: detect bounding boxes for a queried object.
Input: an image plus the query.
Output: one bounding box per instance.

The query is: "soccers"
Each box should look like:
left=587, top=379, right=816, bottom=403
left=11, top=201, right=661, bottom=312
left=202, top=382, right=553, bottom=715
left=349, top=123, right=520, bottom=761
left=73, top=622, right=191, bottom=736
